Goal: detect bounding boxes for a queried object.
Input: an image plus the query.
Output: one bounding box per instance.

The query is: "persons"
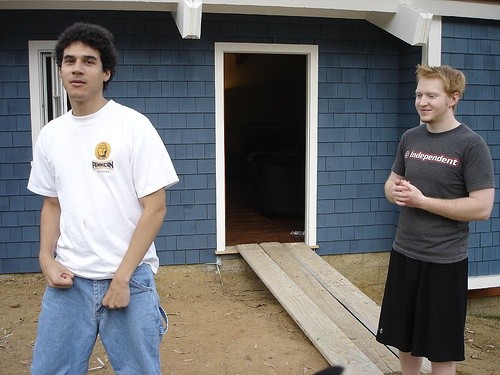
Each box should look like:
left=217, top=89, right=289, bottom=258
left=376, top=63, right=495, bottom=375
left=29, top=23, right=179, bottom=375
